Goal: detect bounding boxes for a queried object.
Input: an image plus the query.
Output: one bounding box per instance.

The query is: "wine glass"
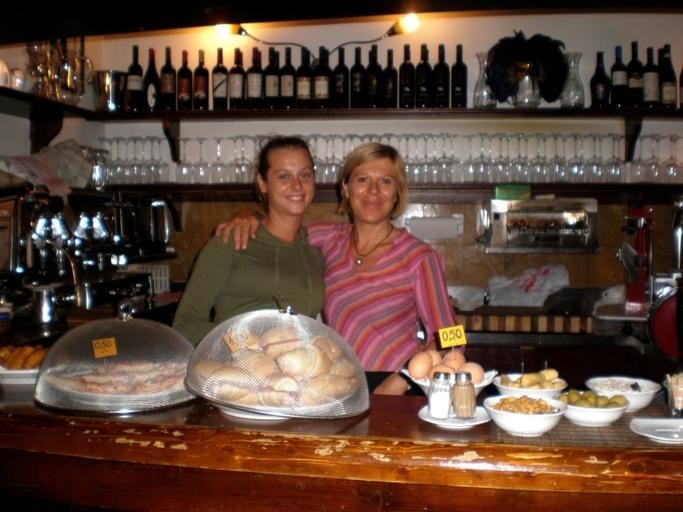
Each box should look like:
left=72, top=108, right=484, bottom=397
left=96, top=133, right=682, bottom=184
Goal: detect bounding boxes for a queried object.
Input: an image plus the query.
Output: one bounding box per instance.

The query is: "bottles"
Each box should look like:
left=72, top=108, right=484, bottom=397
left=428, top=371, right=477, bottom=421
left=126, top=41, right=681, bottom=111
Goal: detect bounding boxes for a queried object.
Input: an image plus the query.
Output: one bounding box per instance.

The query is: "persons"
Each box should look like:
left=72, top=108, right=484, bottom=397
left=212, top=143, right=465, bottom=395
left=171, top=134, right=324, bottom=349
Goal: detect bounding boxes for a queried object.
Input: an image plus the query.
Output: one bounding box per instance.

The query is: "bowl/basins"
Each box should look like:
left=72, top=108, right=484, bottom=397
left=410, top=372, right=492, bottom=399
left=484, top=369, right=661, bottom=440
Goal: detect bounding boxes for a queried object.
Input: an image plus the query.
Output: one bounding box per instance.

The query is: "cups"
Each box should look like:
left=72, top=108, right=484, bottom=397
left=663, top=374, right=683, bottom=418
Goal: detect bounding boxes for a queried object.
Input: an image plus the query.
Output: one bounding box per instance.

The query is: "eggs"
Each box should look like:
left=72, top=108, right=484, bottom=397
left=408, top=349, right=484, bottom=385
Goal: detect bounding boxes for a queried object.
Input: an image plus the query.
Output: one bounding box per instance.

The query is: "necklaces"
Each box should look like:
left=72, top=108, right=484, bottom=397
left=352, top=223, right=393, bottom=265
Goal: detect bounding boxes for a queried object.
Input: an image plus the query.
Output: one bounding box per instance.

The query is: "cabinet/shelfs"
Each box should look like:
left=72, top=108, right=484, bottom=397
left=0, top=85, right=104, bottom=203
left=83, top=108, right=683, bottom=232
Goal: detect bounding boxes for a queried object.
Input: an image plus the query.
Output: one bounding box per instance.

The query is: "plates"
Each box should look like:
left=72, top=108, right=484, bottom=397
left=418, top=405, right=493, bottom=431
left=631, top=418, right=683, bottom=444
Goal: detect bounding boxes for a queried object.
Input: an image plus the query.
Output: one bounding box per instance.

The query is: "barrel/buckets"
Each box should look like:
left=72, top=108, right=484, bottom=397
left=86, top=68, right=128, bottom=111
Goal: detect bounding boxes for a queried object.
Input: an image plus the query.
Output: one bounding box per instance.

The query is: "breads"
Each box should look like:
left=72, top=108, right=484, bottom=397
left=501, top=369, right=566, bottom=391
left=0, top=345, right=188, bottom=394
left=193, top=325, right=363, bottom=407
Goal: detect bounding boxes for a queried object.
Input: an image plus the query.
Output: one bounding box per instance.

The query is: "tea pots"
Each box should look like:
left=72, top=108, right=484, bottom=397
left=60, top=50, right=95, bottom=107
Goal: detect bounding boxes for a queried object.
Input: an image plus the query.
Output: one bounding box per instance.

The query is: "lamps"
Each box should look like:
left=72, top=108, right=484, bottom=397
left=216, top=11, right=421, bottom=60
